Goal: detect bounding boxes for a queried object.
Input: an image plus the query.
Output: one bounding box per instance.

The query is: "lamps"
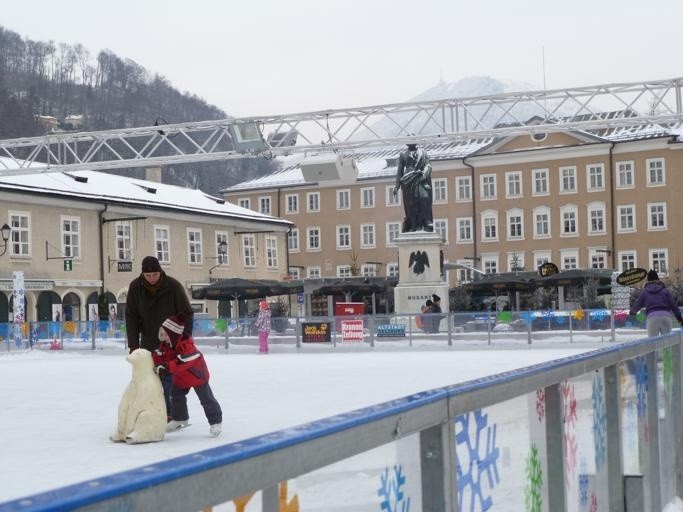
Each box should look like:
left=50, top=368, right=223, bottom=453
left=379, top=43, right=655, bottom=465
left=209, top=241, right=227, bottom=274
left=0, top=223, right=11, bottom=257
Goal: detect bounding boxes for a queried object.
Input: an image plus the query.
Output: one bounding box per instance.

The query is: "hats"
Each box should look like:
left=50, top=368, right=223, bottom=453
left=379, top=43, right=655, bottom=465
left=159, top=312, right=185, bottom=348
left=647, top=269, right=658, bottom=281
left=426, top=294, right=440, bottom=306
left=142, top=256, right=161, bottom=272
left=258, top=301, right=269, bottom=311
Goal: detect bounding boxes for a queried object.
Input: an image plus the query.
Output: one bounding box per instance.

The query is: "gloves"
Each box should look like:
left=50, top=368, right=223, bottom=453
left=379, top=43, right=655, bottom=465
left=677, top=316, right=683, bottom=326
left=629, top=310, right=637, bottom=320
left=153, top=365, right=166, bottom=375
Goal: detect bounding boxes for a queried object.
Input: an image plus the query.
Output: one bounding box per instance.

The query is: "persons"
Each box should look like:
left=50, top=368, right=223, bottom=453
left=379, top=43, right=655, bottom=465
left=393, top=133, right=433, bottom=232
left=112, top=256, right=225, bottom=436
left=254, top=300, right=272, bottom=353
left=416, top=294, right=447, bottom=333
left=629, top=270, right=683, bottom=336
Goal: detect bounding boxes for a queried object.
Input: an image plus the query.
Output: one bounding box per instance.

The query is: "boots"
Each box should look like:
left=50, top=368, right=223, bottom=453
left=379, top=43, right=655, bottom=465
left=166, top=419, right=188, bottom=432
left=210, top=422, right=221, bottom=435
